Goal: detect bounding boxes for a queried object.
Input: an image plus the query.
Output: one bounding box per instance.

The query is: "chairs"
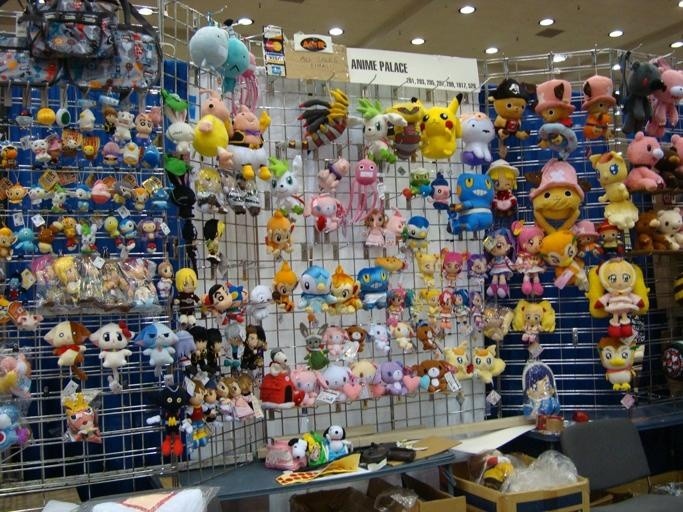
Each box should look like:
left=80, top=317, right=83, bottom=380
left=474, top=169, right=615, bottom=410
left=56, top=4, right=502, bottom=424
left=560, top=416, right=683, bottom=512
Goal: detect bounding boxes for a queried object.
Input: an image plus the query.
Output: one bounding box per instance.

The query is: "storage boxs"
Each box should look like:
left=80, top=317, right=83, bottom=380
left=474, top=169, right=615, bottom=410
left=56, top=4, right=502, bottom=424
left=288, top=480, right=370, bottom=511
left=439, top=453, right=589, bottom=512
left=369, top=472, right=466, bottom=512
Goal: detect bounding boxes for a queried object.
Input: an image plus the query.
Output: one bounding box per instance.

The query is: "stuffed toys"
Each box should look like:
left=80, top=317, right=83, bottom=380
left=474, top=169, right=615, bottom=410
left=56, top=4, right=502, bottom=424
left=597, top=337, right=637, bottom=392
